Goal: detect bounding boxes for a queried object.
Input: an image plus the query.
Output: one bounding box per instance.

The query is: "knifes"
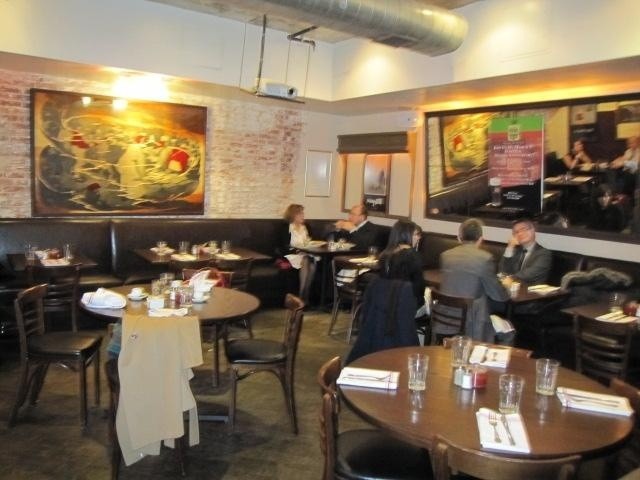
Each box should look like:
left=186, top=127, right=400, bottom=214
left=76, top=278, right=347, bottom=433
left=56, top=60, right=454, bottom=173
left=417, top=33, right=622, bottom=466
left=480, top=348, right=489, bottom=364
left=500, top=414, right=515, bottom=445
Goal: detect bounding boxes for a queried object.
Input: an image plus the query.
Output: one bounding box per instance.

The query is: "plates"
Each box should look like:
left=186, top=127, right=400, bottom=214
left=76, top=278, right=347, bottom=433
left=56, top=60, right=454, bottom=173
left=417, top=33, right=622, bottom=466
left=191, top=296, right=209, bottom=303
left=308, top=241, right=325, bottom=247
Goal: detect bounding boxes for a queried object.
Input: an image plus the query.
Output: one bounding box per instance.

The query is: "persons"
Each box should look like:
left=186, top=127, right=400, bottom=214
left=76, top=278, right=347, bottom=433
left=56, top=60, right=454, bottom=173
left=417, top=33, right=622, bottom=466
left=376, top=216, right=430, bottom=319
left=560, top=136, right=594, bottom=175
left=275, top=202, right=318, bottom=312
left=498, top=217, right=560, bottom=289
left=583, top=181, right=626, bottom=235
left=434, top=219, right=518, bottom=347
left=609, top=134, right=640, bottom=194
left=320, top=201, right=380, bottom=314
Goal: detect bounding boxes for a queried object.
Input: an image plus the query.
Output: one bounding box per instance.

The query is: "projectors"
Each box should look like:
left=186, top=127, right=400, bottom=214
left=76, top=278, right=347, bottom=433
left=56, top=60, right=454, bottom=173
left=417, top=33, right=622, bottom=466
left=256, top=78, right=297, bottom=99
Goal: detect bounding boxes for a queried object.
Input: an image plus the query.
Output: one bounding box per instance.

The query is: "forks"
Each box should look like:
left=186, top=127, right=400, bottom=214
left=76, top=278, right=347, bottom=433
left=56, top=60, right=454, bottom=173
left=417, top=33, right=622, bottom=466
left=487, top=413, right=501, bottom=443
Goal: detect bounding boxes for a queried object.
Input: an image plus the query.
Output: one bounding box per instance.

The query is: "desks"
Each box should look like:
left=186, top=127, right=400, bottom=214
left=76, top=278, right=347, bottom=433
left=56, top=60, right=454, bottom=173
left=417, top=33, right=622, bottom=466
left=471, top=161, right=626, bottom=226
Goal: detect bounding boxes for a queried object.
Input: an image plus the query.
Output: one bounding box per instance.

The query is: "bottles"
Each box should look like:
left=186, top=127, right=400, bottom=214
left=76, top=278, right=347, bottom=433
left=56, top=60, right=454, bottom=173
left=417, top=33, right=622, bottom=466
left=452, top=363, right=473, bottom=390
left=456, top=389, right=477, bottom=412
left=507, top=282, right=520, bottom=291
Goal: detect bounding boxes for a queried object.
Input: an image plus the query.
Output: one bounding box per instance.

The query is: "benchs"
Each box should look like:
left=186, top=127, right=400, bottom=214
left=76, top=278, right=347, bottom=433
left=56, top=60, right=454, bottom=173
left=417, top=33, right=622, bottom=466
left=428, top=151, right=558, bottom=218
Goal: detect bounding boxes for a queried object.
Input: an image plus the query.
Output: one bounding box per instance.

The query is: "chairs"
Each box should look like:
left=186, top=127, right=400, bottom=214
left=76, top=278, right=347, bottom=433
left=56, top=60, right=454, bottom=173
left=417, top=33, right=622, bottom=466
left=1, top=218, right=640, bottom=480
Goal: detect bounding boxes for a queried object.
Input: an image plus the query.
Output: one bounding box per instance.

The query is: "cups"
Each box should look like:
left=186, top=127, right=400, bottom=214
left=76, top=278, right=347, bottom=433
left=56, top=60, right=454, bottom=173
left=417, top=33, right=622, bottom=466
left=156, top=237, right=231, bottom=256
left=449, top=334, right=472, bottom=368
left=49, top=250, right=60, bottom=259
left=127, top=272, right=194, bottom=308
left=328, top=239, right=346, bottom=250
left=407, top=351, right=430, bottom=391
left=24, top=243, right=38, bottom=260
left=536, top=397, right=561, bottom=425
left=608, top=292, right=637, bottom=316
left=497, top=374, right=525, bottom=415
left=62, top=243, right=72, bottom=259
left=407, top=391, right=430, bottom=427
left=469, top=365, right=488, bottom=389
left=368, top=247, right=378, bottom=260
left=535, top=358, right=560, bottom=396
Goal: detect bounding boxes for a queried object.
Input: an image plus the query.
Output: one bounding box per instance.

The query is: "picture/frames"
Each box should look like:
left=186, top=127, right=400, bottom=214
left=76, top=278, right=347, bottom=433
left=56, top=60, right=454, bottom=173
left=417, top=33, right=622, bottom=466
left=29, top=87, right=208, bottom=218
left=438, top=110, right=519, bottom=188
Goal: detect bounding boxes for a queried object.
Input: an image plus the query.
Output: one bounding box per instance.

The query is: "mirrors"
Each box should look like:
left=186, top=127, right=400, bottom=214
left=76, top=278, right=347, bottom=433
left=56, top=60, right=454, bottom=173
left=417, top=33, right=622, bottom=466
left=423, top=91, right=639, bottom=245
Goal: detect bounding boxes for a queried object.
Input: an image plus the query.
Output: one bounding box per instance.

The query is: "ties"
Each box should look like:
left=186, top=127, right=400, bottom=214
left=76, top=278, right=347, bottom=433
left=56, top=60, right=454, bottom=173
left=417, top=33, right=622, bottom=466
left=518, top=249, right=527, bottom=273
